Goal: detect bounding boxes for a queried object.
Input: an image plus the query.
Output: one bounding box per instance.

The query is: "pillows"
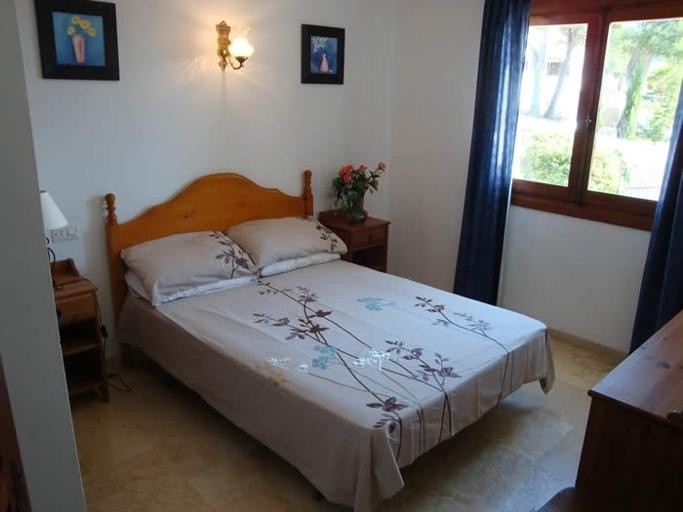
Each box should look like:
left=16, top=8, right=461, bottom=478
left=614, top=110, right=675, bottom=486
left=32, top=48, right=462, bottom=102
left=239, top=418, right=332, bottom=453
left=119, top=216, right=348, bottom=307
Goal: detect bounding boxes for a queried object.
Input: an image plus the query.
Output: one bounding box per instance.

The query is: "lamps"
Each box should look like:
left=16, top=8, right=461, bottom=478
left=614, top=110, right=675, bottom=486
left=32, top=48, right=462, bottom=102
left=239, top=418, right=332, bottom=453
left=215, top=21, right=253, bottom=71
left=40, top=190, right=68, bottom=291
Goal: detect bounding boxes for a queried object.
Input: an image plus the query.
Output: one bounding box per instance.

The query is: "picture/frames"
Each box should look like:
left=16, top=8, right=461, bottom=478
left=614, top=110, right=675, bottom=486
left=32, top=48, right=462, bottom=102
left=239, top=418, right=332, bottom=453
left=301, top=24, right=345, bottom=84
left=35, top=0, right=119, bottom=80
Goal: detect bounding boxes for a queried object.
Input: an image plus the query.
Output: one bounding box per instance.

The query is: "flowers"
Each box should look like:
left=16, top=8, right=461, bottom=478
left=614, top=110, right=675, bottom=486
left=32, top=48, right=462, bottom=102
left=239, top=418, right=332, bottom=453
left=331, top=161, right=386, bottom=211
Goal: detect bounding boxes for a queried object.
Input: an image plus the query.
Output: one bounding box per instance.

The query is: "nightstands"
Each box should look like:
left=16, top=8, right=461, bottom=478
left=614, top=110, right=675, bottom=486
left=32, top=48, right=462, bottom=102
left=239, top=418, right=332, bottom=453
left=319, top=207, right=391, bottom=272
left=50, top=257, right=110, bottom=404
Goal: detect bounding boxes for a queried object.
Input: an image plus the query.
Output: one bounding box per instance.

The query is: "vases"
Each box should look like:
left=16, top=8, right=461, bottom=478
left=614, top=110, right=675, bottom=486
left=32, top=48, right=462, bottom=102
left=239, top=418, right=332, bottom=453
left=345, top=206, right=367, bottom=224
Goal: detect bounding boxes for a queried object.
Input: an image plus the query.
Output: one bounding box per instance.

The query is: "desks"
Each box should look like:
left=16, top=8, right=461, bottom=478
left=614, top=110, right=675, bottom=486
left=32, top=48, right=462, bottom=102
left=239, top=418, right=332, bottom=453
left=574, top=308, right=683, bottom=511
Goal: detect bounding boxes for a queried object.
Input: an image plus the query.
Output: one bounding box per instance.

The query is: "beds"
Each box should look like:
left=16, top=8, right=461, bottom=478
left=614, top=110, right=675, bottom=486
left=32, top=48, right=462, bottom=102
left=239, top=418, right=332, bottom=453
left=101, top=170, right=556, bottom=511
